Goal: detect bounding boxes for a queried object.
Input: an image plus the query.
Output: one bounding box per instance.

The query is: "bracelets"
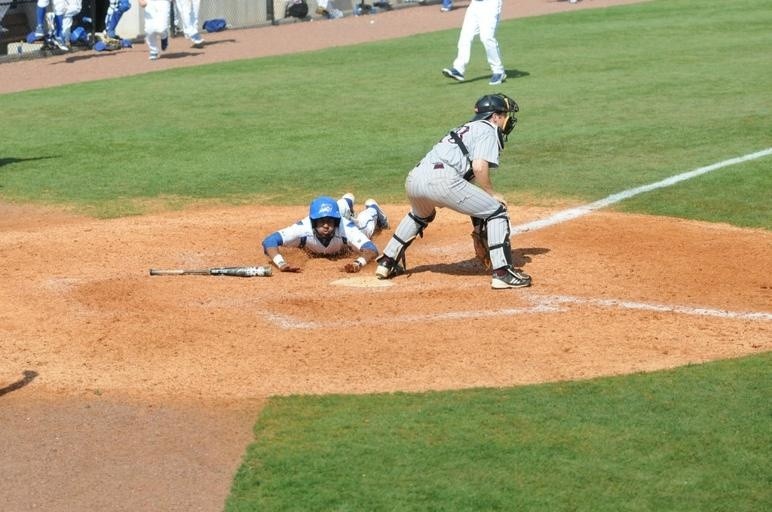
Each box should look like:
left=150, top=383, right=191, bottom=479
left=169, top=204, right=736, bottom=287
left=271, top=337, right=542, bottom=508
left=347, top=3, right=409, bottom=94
left=273, top=254, right=287, bottom=268
left=352, top=256, right=367, bottom=268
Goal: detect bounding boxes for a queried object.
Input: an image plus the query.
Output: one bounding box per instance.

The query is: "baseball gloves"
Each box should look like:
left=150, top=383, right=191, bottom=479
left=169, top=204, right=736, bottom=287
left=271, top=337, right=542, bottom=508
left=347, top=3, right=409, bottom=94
left=473, top=231, right=491, bottom=271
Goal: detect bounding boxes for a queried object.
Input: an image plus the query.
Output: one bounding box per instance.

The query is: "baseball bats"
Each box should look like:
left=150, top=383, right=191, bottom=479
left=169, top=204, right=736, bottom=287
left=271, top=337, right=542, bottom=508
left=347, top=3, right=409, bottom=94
left=150, top=265, right=272, bottom=277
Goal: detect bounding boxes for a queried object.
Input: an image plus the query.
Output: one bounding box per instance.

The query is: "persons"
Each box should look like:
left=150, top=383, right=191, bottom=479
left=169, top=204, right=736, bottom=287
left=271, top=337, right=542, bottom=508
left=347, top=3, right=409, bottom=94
left=262, top=191, right=389, bottom=274
left=27, top=0, right=206, bottom=61
left=441, top=0, right=506, bottom=87
left=375, top=93, right=532, bottom=290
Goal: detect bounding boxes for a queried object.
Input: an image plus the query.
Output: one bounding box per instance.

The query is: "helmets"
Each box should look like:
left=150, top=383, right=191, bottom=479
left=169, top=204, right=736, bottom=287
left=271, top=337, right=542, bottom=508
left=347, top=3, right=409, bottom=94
left=469, top=93, right=518, bottom=152
left=309, top=196, right=341, bottom=222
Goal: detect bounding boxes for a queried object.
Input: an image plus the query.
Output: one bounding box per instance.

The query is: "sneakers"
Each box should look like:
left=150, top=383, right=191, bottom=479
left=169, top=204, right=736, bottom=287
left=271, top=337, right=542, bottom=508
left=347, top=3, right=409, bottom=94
left=491, top=271, right=530, bottom=288
left=342, top=193, right=354, bottom=217
left=365, top=199, right=390, bottom=230
left=375, top=260, right=404, bottom=277
left=442, top=68, right=464, bottom=81
left=489, top=73, right=507, bottom=85
